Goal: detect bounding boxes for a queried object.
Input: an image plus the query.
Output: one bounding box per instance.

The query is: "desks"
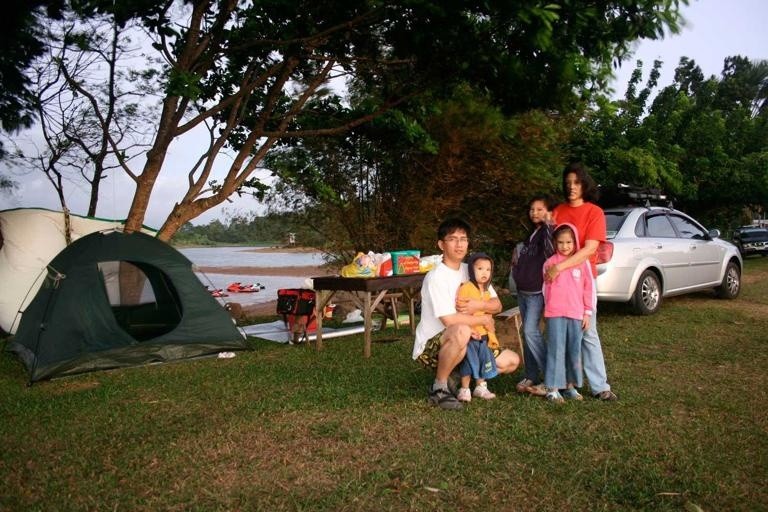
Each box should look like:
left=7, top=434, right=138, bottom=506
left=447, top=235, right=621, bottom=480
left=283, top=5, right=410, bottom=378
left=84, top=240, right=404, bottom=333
left=311, top=271, right=429, bottom=357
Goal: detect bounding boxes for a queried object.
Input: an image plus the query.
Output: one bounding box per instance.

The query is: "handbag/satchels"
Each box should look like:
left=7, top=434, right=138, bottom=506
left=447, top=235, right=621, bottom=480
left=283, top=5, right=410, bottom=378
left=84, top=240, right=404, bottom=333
left=276, top=288, right=317, bottom=317
left=314, top=288, right=331, bottom=306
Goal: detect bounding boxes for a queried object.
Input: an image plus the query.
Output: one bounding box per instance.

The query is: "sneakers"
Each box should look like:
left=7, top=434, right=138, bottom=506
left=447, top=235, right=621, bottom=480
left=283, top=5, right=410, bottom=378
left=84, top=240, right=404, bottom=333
left=595, top=391, right=617, bottom=401
left=516, top=377, right=550, bottom=395
left=473, top=381, right=496, bottom=399
left=545, top=392, right=565, bottom=402
left=563, top=388, right=584, bottom=401
left=428, top=372, right=472, bottom=409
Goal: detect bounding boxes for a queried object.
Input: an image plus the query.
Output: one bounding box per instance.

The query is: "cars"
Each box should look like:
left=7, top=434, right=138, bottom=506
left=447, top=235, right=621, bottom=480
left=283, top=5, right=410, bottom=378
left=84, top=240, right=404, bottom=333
left=507, top=205, right=742, bottom=314
left=730, top=224, right=767, bottom=260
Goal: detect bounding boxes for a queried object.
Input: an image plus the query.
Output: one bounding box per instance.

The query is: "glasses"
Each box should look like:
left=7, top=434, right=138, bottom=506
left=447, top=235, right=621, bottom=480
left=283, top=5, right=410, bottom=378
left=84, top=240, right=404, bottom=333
left=444, top=237, right=470, bottom=243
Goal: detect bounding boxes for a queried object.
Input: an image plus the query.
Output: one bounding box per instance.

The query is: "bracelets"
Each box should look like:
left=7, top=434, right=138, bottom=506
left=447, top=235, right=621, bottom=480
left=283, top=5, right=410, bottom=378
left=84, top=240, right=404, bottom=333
left=481, top=300, right=488, bottom=312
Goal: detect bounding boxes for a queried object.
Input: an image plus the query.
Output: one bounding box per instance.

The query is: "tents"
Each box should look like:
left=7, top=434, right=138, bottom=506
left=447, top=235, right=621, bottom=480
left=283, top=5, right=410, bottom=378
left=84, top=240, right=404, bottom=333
left=0, top=226, right=257, bottom=389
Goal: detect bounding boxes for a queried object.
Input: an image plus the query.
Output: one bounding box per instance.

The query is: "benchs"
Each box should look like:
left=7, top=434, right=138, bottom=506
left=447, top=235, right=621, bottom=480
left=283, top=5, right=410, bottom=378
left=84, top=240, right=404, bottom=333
left=493, top=305, right=546, bottom=367
left=330, top=292, right=404, bottom=331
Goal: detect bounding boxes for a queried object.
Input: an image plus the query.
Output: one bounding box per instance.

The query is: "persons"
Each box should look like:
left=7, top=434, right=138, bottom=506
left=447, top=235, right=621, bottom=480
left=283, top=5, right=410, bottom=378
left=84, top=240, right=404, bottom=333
left=539, top=224, right=597, bottom=404
left=411, top=217, right=521, bottom=412
left=545, top=164, right=618, bottom=402
left=455, top=252, right=499, bottom=402
left=510, top=191, right=557, bottom=395
